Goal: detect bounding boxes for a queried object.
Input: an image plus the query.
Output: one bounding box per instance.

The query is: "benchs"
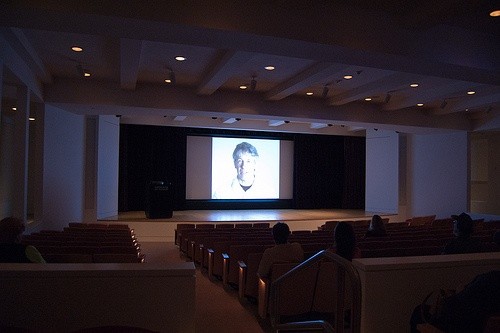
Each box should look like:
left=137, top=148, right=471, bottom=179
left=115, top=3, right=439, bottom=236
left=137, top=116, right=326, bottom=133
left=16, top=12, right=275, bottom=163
left=1, top=216, right=197, bottom=333
left=174, top=215, right=500, bottom=333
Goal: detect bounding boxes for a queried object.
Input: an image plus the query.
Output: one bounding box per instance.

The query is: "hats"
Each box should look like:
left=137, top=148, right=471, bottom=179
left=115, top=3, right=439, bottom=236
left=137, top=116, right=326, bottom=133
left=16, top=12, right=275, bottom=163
left=451, top=212, right=473, bottom=224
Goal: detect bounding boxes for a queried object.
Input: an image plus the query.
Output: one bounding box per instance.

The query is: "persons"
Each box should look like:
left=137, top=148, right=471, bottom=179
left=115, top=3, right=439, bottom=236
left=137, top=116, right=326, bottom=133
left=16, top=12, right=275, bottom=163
left=367, top=215, right=385, bottom=236
left=0, top=217, right=46, bottom=264
left=444, top=213, right=479, bottom=255
left=213, top=142, right=278, bottom=199
left=326, top=221, right=360, bottom=262
left=258, top=222, right=304, bottom=275
